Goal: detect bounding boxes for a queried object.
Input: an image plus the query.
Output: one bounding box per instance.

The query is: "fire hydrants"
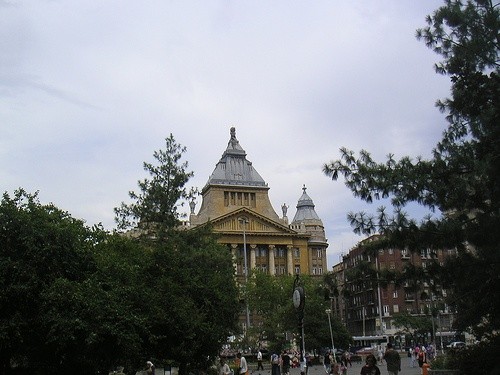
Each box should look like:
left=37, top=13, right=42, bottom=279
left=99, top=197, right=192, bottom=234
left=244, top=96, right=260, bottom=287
left=422, top=363, right=429, bottom=375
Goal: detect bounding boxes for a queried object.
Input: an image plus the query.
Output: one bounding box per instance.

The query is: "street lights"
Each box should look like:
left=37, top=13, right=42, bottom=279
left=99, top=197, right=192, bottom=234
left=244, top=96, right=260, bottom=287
left=325, top=309, right=337, bottom=365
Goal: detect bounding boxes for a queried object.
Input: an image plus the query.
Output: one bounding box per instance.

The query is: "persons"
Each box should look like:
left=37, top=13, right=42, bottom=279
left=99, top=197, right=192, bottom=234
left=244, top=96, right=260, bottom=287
left=218, top=342, right=435, bottom=375
left=144, top=359, right=155, bottom=375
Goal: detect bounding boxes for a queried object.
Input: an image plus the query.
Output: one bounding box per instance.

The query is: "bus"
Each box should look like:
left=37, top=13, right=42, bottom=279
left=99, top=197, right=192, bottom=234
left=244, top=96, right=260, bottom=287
left=350, top=333, right=471, bottom=354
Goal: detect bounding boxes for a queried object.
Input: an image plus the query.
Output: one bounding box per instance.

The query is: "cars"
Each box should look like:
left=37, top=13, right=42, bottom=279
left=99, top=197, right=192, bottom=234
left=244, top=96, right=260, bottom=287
left=451, top=342, right=467, bottom=350
left=355, top=348, right=375, bottom=355
left=327, top=349, right=344, bottom=355
